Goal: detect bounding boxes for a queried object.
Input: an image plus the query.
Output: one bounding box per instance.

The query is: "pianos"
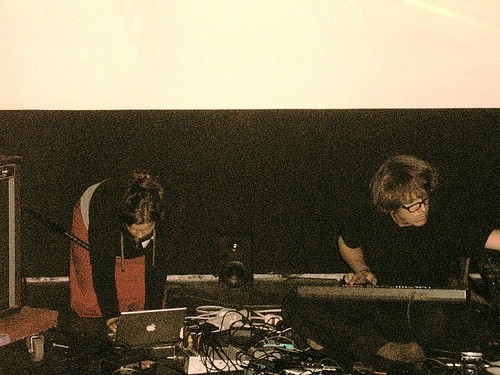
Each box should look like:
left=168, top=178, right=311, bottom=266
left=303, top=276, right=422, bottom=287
left=284, top=277, right=471, bottom=303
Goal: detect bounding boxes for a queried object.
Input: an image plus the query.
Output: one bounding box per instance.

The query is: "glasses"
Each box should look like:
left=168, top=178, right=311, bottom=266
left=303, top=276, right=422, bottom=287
left=401, top=197, right=430, bottom=212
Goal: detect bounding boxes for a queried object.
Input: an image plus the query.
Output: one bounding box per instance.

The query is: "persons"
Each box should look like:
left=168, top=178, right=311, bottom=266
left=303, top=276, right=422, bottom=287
left=335, top=150, right=499, bottom=340
left=66, top=167, right=175, bottom=341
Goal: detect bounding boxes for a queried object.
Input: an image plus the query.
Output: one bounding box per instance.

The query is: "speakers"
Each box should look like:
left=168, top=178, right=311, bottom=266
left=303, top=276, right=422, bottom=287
left=217, top=233, right=255, bottom=305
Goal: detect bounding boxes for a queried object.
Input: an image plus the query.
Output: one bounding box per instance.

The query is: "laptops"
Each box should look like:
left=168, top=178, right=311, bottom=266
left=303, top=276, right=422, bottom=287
left=108, top=306, right=188, bottom=347
